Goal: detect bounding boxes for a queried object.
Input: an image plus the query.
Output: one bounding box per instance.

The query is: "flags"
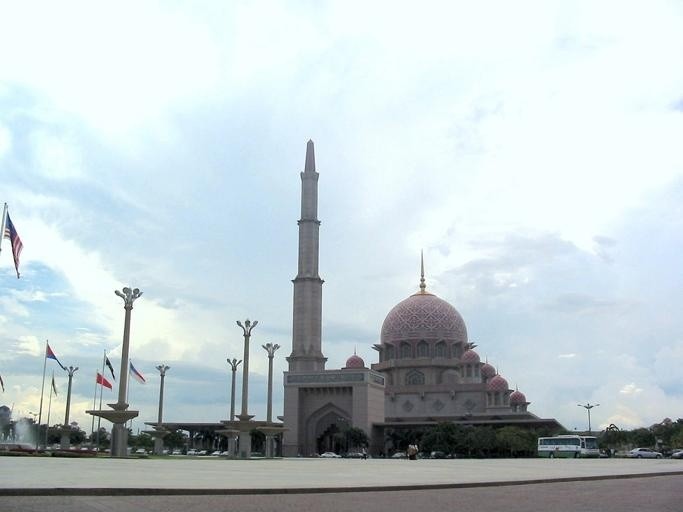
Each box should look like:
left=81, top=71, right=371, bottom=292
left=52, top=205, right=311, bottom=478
left=52, top=379, right=57, bottom=397
left=106, top=358, right=115, bottom=380
left=48, top=344, right=63, bottom=370
left=131, top=361, right=146, bottom=384
left=97, top=373, right=112, bottom=389
left=4, top=211, right=24, bottom=278
left=0, top=376, right=5, bottom=392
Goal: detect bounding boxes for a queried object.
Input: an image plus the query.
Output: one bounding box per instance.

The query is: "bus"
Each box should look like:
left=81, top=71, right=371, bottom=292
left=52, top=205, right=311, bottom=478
left=537, top=434, right=599, bottom=458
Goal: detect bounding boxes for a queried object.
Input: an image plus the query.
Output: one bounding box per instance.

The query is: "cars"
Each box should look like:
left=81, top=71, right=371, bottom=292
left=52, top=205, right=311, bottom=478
left=28, top=443, right=228, bottom=455
left=320, top=451, right=341, bottom=458
left=345, top=453, right=369, bottom=459
left=628, top=447, right=662, bottom=458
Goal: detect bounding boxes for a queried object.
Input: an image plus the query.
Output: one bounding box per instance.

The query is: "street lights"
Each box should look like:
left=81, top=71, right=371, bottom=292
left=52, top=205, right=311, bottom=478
left=237, top=320, right=258, bottom=414
left=115, top=287, right=143, bottom=401
left=64, top=366, right=79, bottom=425
left=29, top=412, right=40, bottom=423
left=156, top=365, right=170, bottom=424
left=577, top=404, right=600, bottom=433
left=261, top=343, right=280, bottom=422
left=227, top=358, right=243, bottom=422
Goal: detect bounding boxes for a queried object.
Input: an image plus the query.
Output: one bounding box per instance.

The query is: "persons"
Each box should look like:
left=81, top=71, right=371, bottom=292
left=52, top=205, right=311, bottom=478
left=360, top=447, right=367, bottom=461
left=407, top=444, right=418, bottom=460
